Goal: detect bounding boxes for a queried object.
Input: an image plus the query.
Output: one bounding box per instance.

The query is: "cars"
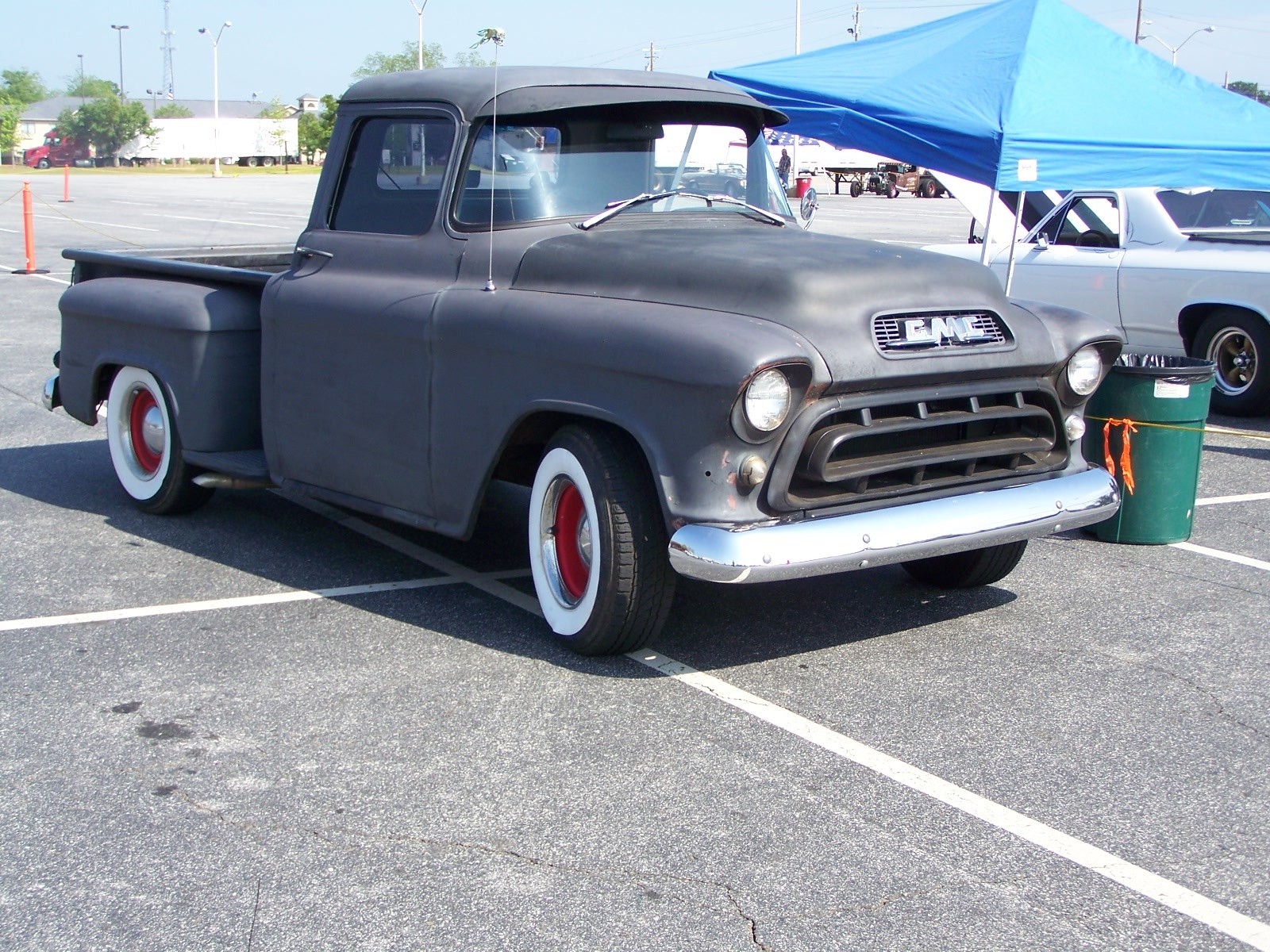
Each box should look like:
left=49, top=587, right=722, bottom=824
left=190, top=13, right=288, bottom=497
left=917, top=187, right=1270, bottom=417
left=680, top=163, right=789, bottom=199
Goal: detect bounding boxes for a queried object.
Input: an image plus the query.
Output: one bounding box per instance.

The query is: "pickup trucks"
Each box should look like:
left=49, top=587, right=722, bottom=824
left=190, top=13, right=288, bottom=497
left=40, top=65, right=1122, bottom=657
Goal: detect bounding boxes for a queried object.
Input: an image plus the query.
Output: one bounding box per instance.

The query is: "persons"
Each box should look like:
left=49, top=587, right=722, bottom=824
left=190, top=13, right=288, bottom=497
left=779, top=148, right=791, bottom=188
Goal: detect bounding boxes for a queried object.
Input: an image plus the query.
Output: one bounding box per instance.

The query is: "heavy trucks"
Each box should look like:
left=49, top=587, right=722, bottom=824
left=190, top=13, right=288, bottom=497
left=23, top=116, right=300, bottom=169
left=450, top=126, right=945, bottom=218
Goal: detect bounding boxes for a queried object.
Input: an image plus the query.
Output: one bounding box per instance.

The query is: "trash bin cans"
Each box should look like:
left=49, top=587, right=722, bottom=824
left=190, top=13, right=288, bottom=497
left=1080, top=355, right=1217, bottom=546
left=796, top=177, right=811, bottom=198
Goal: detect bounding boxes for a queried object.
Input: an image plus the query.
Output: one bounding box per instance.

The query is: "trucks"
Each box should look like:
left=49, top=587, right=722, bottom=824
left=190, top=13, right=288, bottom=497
left=849, top=161, right=954, bottom=199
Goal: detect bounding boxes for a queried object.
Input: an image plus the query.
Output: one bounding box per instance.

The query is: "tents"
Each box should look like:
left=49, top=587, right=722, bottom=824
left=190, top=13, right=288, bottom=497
left=664, top=2, right=1269, bottom=298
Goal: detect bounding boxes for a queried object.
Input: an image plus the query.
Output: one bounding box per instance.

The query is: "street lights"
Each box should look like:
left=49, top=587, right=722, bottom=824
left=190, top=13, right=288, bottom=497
left=197, top=21, right=231, bottom=177
left=76, top=54, right=86, bottom=107
left=1136, top=24, right=1214, bottom=67
left=111, top=24, right=129, bottom=102
